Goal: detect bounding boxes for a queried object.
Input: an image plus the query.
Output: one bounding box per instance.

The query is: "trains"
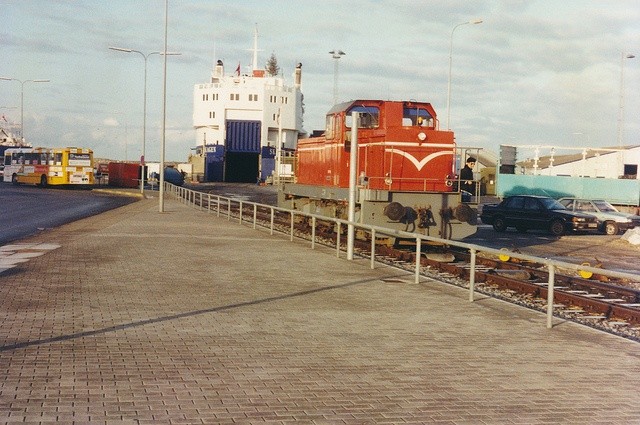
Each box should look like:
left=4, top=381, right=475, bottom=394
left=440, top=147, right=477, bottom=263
left=294, top=99, right=456, bottom=193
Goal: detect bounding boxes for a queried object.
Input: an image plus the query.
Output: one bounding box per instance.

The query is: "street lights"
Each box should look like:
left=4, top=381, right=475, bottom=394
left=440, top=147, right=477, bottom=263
left=108, top=45, right=182, bottom=195
left=617, top=51, right=636, bottom=177
left=0, top=76, right=50, bottom=144
left=444, top=18, right=484, bottom=130
left=329, top=48, right=347, bottom=106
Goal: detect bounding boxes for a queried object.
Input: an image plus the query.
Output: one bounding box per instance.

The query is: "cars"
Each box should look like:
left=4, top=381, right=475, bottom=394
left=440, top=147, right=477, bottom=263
left=479, top=194, right=598, bottom=236
left=97, top=163, right=109, bottom=174
left=548, top=196, right=640, bottom=235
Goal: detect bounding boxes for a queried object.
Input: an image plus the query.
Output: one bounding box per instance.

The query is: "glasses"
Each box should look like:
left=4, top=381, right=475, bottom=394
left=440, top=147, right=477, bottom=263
left=469, top=162, right=476, bottom=165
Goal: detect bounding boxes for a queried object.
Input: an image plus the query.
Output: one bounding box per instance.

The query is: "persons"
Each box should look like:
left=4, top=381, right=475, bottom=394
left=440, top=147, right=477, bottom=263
left=461, top=157, right=477, bottom=193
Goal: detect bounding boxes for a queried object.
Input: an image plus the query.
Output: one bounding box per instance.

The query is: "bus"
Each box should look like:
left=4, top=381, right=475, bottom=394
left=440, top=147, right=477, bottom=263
left=3, top=145, right=94, bottom=189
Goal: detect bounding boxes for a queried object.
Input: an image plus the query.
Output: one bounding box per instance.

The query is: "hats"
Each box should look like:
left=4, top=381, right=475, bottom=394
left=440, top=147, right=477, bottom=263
left=467, top=156, right=476, bottom=162
